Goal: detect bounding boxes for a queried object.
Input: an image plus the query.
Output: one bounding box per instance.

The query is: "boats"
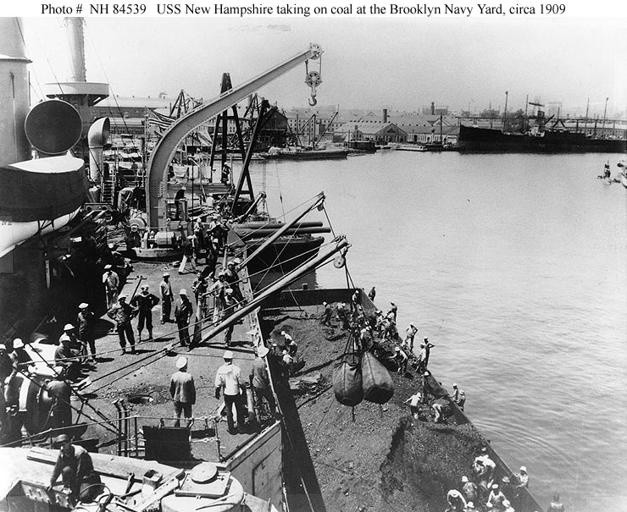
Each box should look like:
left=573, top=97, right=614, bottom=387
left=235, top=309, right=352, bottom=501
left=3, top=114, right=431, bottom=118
left=254, top=285, right=543, bottom=511
left=259, top=147, right=282, bottom=160
left=456, top=92, right=627, bottom=152
left=207, top=193, right=330, bottom=277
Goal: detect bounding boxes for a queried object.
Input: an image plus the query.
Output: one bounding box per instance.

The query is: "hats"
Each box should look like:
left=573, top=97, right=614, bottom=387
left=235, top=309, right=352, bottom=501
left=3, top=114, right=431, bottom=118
left=56, top=434, right=69, bottom=443
left=104, top=265, right=112, bottom=270
left=258, top=346, right=269, bottom=358
left=64, top=324, right=75, bottom=331
left=0, top=338, right=25, bottom=349
left=118, top=296, right=126, bottom=301
left=52, top=366, right=65, bottom=379
left=223, top=351, right=233, bottom=359
left=176, top=357, right=187, bottom=370
left=141, top=285, right=149, bottom=288
left=218, top=258, right=240, bottom=293
left=178, top=289, right=187, bottom=295
left=61, top=336, right=71, bottom=342
left=162, top=273, right=170, bottom=277
left=79, top=303, right=89, bottom=308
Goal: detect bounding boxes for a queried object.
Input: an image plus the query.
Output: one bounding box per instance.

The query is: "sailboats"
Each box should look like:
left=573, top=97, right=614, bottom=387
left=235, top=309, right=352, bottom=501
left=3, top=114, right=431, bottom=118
left=277, top=112, right=349, bottom=161
left=424, top=112, right=465, bottom=152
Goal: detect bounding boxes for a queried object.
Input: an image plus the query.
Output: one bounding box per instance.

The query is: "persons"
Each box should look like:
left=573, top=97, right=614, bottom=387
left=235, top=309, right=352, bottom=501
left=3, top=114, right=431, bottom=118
left=0, top=162, right=564, bottom=512
